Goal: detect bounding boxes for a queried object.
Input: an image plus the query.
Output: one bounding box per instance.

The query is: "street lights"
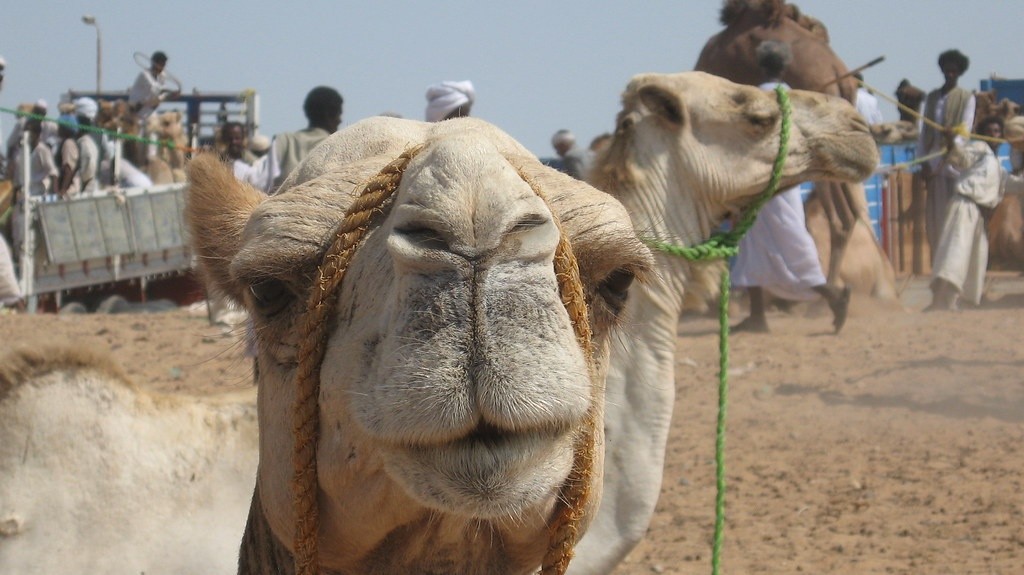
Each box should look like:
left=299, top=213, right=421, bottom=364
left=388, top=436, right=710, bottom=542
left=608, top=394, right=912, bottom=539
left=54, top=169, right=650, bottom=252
left=80, top=13, right=102, bottom=98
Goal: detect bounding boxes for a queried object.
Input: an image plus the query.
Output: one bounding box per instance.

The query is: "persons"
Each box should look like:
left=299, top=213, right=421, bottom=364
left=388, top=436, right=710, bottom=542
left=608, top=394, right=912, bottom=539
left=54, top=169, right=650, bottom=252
left=850, top=72, right=883, bottom=127
left=918, top=116, right=1024, bottom=314
left=536, top=129, right=590, bottom=181
left=231, top=84, right=344, bottom=195
left=721, top=76, right=850, bottom=333
left=127, top=50, right=170, bottom=157
left=0, top=97, right=274, bottom=332
left=424, top=79, right=475, bottom=123
left=917, top=47, right=976, bottom=273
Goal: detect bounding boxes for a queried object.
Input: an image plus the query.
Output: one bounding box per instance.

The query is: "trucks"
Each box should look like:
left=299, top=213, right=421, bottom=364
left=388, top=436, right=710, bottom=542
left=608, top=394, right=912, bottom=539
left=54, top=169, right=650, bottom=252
left=0, top=87, right=276, bottom=314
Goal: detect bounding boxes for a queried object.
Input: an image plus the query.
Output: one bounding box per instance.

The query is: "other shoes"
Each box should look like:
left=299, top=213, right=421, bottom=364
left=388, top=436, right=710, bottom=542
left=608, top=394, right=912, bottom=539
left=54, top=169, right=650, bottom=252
left=728, top=314, right=773, bottom=333
left=829, top=287, right=850, bottom=333
left=956, top=297, right=995, bottom=309
left=920, top=299, right=960, bottom=313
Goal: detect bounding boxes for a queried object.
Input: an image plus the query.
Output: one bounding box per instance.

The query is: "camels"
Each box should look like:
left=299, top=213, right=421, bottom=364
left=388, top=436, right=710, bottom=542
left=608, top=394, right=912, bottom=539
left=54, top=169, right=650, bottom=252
left=182, top=114, right=696, bottom=574
left=0, top=0, right=1024, bottom=573
